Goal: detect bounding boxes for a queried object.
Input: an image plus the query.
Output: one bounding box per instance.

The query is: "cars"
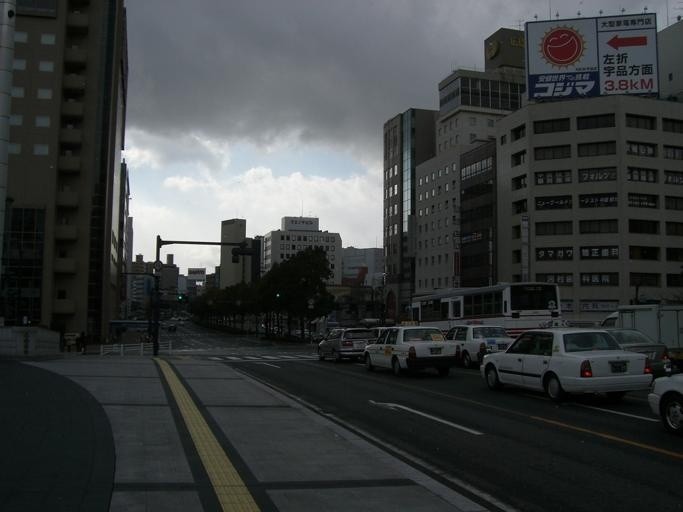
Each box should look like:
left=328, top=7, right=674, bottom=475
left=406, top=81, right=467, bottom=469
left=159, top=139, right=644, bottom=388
left=368, top=326, right=388, bottom=344
left=479, top=327, right=656, bottom=405
left=444, top=324, right=516, bottom=369
left=646, top=374, right=683, bottom=432
left=159, top=316, right=190, bottom=332
left=318, top=327, right=372, bottom=365
left=363, top=326, right=457, bottom=378
left=604, top=328, right=672, bottom=380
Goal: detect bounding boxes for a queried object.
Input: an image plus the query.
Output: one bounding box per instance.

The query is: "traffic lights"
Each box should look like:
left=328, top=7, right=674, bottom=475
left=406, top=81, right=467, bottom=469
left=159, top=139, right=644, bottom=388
left=176, top=295, right=185, bottom=302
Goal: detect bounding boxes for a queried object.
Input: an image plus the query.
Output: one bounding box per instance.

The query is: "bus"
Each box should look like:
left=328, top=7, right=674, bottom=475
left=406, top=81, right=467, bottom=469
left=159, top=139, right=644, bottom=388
left=108, top=318, right=160, bottom=334
left=411, top=281, right=562, bottom=335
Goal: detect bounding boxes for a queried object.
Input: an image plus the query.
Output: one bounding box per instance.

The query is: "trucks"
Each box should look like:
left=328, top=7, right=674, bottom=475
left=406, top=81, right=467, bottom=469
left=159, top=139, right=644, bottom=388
left=598, top=304, right=680, bottom=363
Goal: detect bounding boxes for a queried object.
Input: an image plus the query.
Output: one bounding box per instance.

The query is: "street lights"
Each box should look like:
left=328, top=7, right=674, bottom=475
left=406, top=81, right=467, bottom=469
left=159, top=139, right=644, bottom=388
left=2, top=195, right=14, bottom=291
